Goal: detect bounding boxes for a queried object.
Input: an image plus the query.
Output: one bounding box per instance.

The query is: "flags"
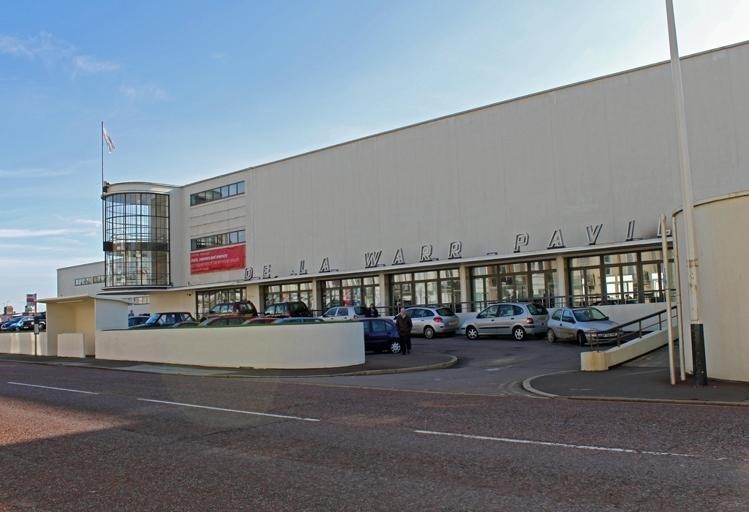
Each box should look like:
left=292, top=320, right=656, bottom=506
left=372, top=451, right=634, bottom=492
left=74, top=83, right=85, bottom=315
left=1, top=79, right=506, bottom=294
left=103, top=126, right=117, bottom=154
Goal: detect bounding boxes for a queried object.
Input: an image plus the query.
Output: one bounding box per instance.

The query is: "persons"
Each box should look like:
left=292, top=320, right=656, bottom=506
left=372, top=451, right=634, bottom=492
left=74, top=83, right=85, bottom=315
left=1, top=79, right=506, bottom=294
left=369, top=303, right=378, bottom=316
left=395, top=306, right=413, bottom=356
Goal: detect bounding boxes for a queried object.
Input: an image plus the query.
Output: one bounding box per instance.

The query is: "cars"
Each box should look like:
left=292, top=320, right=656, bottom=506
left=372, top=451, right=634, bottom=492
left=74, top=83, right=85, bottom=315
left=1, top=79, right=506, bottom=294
left=0, top=315, right=46, bottom=332
